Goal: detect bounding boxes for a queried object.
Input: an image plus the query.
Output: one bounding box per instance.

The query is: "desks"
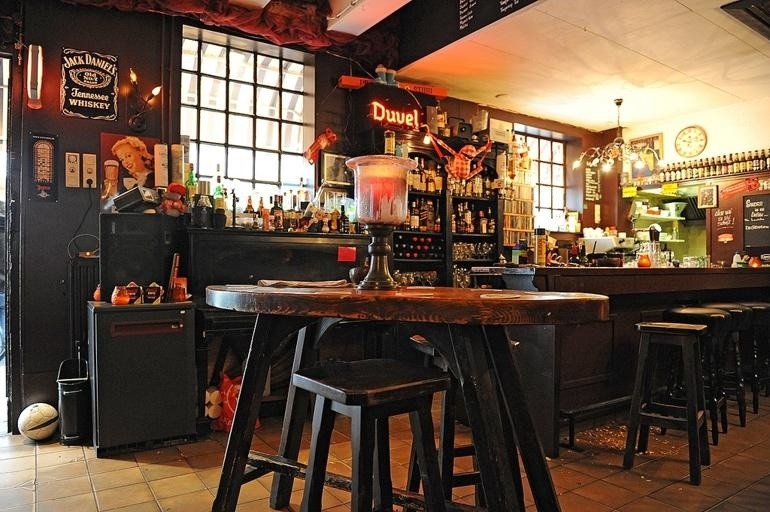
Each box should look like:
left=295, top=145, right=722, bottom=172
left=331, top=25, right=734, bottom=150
left=203, top=281, right=611, bottom=512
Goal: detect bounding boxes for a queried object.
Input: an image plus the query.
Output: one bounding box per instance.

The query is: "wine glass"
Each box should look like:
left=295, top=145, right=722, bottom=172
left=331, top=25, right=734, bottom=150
left=345, top=155, right=418, bottom=291
left=393, top=270, right=438, bottom=286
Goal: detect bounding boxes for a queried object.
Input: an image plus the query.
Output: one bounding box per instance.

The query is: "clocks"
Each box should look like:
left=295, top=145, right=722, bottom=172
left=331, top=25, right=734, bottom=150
left=672, top=122, right=709, bottom=160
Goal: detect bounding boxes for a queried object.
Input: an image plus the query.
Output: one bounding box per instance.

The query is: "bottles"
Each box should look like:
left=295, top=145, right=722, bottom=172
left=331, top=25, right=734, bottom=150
left=258, top=196, right=266, bottom=216
left=186, top=162, right=198, bottom=200
left=683, top=255, right=710, bottom=268
left=285, top=178, right=349, bottom=234
left=214, top=175, right=225, bottom=210
left=633, top=198, right=661, bottom=215
left=244, top=195, right=253, bottom=213
left=384, top=131, right=395, bottom=155
left=407, top=155, right=496, bottom=236
left=535, top=229, right=546, bottom=266
left=603, top=226, right=617, bottom=238
left=443, top=124, right=450, bottom=138
left=166, top=252, right=180, bottom=303
left=732, top=250, right=770, bottom=267
left=111, top=285, right=130, bottom=304
left=638, top=254, right=651, bottom=267
left=173, top=282, right=185, bottom=302
left=658, top=148, right=769, bottom=181
left=263, top=195, right=284, bottom=231
left=433, top=100, right=445, bottom=135
left=214, top=208, right=226, bottom=230
left=197, top=196, right=215, bottom=229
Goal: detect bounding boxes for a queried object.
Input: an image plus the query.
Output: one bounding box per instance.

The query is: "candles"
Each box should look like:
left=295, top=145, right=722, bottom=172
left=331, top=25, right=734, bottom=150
left=366, top=164, right=397, bottom=221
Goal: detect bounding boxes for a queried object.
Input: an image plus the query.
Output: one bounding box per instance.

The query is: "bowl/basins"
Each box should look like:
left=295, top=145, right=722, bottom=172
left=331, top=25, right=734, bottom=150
left=587, top=253, right=624, bottom=266
left=233, top=218, right=254, bottom=231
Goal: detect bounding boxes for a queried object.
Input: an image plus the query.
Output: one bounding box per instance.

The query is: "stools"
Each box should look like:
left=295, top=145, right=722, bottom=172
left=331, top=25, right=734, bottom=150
left=620, top=300, right=770, bottom=488
left=285, top=354, right=459, bottom=512
left=402, top=330, right=524, bottom=512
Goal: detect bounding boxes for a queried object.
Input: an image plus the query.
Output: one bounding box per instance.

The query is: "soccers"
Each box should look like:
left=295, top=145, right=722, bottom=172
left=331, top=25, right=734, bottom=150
left=17, top=403, right=60, bottom=441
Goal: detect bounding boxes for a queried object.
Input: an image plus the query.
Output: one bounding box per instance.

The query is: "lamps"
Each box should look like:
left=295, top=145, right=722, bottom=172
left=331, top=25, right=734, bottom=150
left=123, top=66, right=165, bottom=132
left=569, top=92, right=668, bottom=176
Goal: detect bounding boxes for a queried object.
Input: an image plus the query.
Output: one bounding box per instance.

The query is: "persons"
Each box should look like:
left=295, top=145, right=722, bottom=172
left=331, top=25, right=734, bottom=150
left=111, top=136, right=155, bottom=196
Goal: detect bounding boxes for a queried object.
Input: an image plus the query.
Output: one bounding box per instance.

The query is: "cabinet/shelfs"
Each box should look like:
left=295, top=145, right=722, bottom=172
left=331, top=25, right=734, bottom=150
left=504, top=164, right=536, bottom=249
left=369, top=124, right=507, bottom=287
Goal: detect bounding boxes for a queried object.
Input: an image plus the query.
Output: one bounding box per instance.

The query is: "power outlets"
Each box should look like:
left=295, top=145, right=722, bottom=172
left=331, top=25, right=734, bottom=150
left=82, top=174, right=96, bottom=190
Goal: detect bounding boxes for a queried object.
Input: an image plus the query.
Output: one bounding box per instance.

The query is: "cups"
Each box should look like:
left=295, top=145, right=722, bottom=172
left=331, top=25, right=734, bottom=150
left=103, top=160, right=119, bottom=180
left=673, top=260, right=680, bottom=268
left=649, top=227, right=659, bottom=241
left=375, top=68, right=386, bottom=82
left=559, top=248, right=568, bottom=266
left=386, top=70, right=398, bottom=84
left=198, top=181, right=210, bottom=195
